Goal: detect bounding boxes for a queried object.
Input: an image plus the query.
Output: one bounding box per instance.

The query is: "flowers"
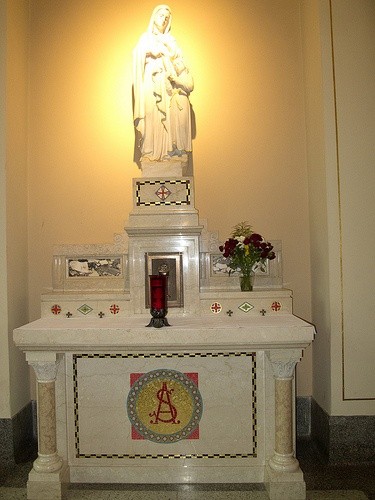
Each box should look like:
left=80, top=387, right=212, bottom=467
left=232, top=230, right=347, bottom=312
left=218, top=218, right=277, bottom=291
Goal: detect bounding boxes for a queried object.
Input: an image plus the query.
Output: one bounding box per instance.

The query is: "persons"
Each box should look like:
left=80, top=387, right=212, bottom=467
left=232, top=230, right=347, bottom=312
left=164, top=56, right=194, bottom=157
left=129, top=3, right=191, bottom=162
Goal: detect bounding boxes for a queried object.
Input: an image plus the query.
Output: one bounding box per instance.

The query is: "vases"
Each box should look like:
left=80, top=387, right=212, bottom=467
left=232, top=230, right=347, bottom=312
left=238, top=265, right=255, bottom=292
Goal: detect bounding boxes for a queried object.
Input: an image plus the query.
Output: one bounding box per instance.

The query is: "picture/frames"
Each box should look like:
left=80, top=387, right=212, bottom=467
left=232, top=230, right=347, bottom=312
left=145, top=251, right=184, bottom=309
